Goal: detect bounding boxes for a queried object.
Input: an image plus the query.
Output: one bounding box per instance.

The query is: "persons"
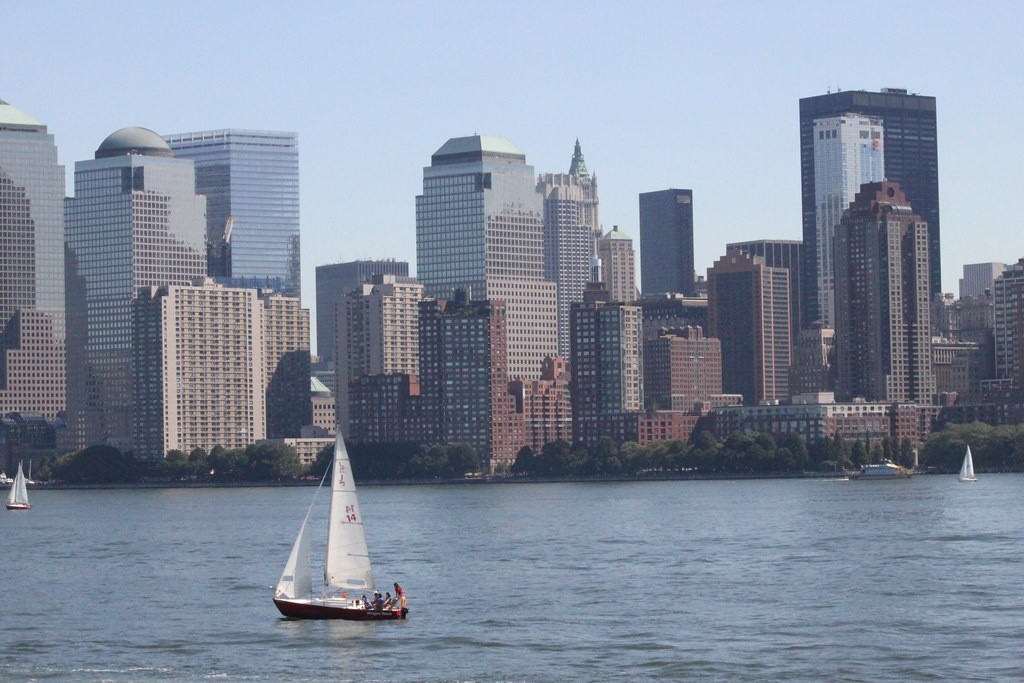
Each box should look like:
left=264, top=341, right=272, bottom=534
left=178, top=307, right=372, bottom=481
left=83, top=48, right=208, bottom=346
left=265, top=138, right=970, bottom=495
left=342, top=591, right=347, bottom=598
left=362, top=583, right=403, bottom=610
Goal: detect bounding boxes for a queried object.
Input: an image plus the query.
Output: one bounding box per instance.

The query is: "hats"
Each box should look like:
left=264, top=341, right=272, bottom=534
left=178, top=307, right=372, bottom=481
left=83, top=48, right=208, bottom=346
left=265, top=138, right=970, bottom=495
left=373, top=591, right=379, bottom=596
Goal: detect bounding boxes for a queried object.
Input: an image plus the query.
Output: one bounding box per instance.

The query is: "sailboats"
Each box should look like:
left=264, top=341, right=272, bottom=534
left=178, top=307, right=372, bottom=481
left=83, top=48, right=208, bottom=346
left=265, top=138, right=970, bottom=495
left=5, top=458, right=33, bottom=510
left=956, top=444, right=979, bottom=483
left=272, top=421, right=404, bottom=621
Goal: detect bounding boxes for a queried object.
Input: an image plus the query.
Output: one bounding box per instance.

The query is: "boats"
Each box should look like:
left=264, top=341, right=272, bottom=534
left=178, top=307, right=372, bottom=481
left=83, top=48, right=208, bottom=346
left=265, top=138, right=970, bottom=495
left=846, top=456, right=914, bottom=481
